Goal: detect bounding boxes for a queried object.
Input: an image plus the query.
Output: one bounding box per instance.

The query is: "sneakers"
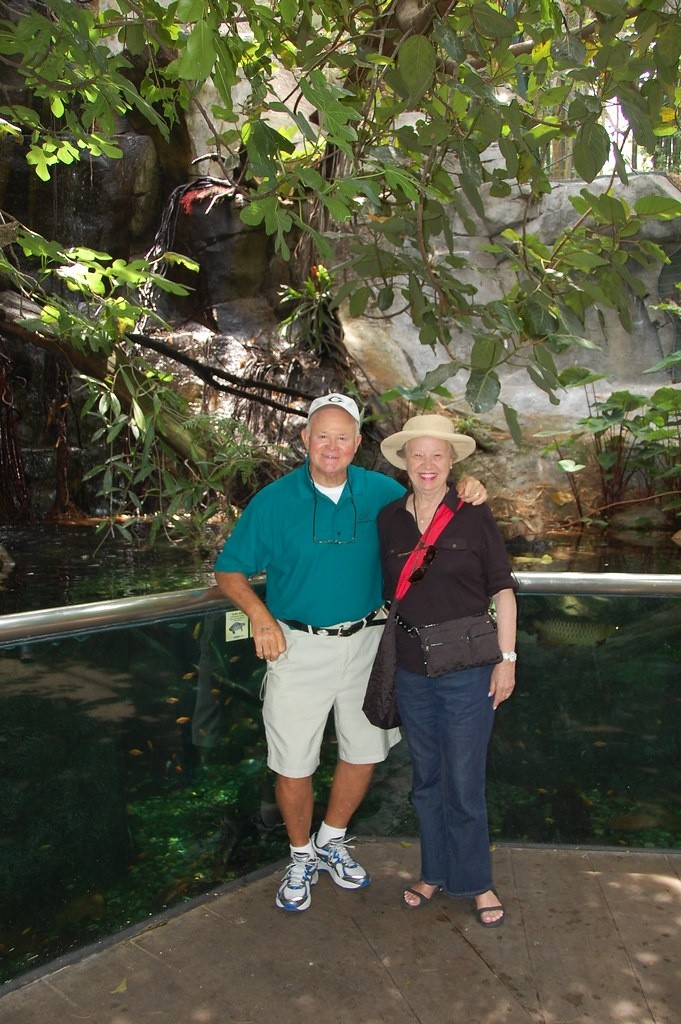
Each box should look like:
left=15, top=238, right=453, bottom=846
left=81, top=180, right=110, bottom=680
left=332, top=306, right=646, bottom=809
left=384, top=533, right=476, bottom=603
left=311, top=832, right=371, bottom=889
left=275, top=852, right=321, bottom=913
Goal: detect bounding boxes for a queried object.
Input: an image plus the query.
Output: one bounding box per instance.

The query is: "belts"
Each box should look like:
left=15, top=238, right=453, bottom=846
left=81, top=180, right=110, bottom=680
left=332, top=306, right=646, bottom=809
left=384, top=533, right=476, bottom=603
left=278, top=609, right=387, bottom=637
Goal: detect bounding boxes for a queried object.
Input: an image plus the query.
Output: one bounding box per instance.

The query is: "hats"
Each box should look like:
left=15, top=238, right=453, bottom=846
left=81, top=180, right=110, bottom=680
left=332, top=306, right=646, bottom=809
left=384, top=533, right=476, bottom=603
left=308, top=392, right=361, bottom=429
left=380, top=415, right=476, bottom=470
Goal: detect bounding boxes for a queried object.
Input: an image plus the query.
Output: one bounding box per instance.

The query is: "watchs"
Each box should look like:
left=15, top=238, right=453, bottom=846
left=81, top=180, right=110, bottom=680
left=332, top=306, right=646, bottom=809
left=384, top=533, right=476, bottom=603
left=503, top=653, right=517, bottom=662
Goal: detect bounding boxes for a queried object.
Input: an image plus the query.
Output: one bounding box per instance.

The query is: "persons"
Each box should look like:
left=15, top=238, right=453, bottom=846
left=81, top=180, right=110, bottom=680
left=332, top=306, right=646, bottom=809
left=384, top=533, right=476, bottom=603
left=374, top=415, right=518, bottom=928
left=214, top=393, right=491, bottom=912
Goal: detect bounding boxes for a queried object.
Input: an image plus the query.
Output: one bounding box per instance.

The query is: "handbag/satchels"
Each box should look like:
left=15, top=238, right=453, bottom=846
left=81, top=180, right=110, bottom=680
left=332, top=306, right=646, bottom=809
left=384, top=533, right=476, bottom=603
left=362, top=620, right=402, bottom=730
left=418, top=612, right=503, bottom=680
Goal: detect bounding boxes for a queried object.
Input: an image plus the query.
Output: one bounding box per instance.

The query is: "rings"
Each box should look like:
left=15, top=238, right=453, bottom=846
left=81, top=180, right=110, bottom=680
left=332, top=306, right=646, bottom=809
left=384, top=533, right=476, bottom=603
left=475, top=492, right=481, bottom=496
left=506, top=694, right=511, bottom=698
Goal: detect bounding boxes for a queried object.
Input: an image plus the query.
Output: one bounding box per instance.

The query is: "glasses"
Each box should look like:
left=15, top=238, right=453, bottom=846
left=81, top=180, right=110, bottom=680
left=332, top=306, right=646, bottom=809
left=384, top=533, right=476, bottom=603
left=312, top=495, right=356, bottom=544
left=396, top=540, right=439, bottom=586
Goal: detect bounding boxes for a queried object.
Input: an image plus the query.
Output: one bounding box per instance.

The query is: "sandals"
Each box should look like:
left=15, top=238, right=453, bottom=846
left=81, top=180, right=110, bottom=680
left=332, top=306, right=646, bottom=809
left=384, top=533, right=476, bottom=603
left=472, top=887, right=505, bottom=929
left=401, top=881, right=445, bottom=911
